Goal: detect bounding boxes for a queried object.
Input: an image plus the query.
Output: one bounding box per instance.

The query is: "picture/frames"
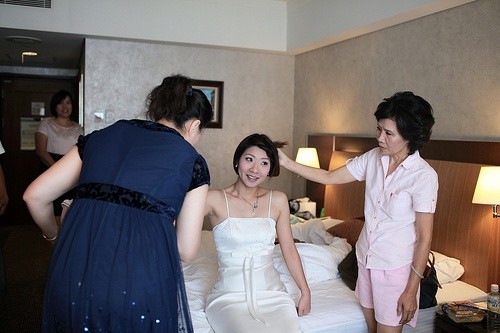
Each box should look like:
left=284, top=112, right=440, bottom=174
left=188, top=79, right=223, bottom=129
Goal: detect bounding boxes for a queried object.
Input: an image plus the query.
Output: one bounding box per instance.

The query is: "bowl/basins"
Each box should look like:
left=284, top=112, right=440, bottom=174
left=439, top=300, right=488, bottom=323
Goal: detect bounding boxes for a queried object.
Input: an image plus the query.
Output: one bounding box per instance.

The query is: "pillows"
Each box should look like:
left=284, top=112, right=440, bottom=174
left=427, top=250, right=465, bottom=284
left=326, top=218, right=364, bottom=248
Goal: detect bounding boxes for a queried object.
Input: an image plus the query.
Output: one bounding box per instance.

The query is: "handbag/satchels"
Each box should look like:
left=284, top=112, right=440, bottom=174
left=337, top=248, right=358, bottom=291
left=288, top=197, right=316, bottom=218
left=419, top=251, right=443, bottom=309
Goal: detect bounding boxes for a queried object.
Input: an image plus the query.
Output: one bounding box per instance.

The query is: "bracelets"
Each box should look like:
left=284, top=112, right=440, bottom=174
left=43, top=232, right=57, bottom=241
left=411, top=265, right=424, bottom=279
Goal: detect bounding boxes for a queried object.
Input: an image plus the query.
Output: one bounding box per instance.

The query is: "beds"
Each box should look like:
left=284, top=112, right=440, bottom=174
left=181, top=135, right=500, bottom=333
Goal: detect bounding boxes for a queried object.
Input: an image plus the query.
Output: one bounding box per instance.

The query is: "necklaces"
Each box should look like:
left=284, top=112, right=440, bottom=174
left=234, top=183, right=259, bottom=217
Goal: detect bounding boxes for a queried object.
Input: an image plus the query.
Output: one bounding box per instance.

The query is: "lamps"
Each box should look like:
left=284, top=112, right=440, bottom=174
left=294, top=147, right=320, bottom=168
left=471, top=166, right=500, bottom=218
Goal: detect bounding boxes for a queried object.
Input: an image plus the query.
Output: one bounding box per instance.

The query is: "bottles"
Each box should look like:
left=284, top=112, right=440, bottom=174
left=486, top=284, right=500, bottom=333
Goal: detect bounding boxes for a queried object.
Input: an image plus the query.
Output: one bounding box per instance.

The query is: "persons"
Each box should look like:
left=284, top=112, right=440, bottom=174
left=59, top=199, right=74, bottom=222
left=277, top=90, right=439, bottom=333
left=34, top=89, right=84, bottom=215
left=23, top=72, right=215, bottom=333
left=0, top=138, right=9, bottom=307
left=172, top=132, right=311, bottom=333
